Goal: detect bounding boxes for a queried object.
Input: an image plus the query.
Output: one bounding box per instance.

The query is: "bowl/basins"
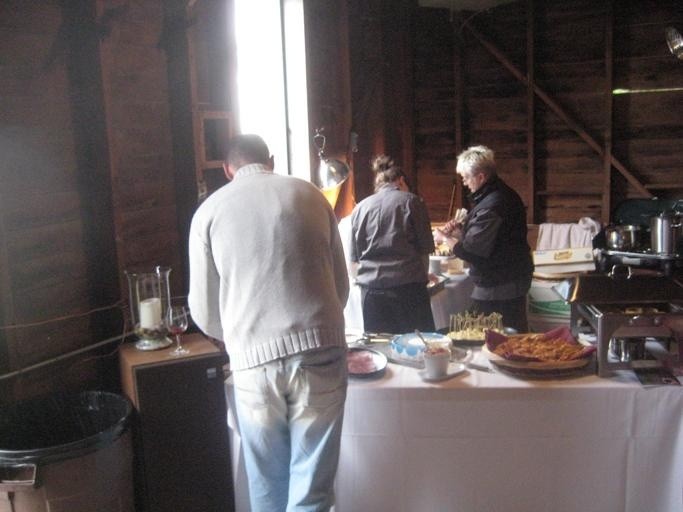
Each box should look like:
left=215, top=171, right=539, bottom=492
left=391, top=330, right=455, bottom=360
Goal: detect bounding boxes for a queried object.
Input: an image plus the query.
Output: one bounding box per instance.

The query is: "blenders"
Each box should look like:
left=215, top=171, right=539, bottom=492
left=124, top=266, right=174, bottom=351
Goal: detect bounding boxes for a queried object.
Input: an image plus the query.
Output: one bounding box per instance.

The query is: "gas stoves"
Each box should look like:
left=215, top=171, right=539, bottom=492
left=600, top=246, right=677, bottom=259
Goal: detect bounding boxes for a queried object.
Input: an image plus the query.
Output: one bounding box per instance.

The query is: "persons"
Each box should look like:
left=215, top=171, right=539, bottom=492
left=187, top=135, right=349, bottom=511
left=433, top=144, right=534, bottom=334
left=348, top=152, right=435, bottom=333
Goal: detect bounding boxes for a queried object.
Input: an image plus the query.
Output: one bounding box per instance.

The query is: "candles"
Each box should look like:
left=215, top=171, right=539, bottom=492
left=138, top=297, right=161, bottom=328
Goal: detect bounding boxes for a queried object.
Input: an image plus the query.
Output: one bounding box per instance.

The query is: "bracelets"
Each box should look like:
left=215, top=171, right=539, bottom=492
left=442, top=236, right=450, bottom=246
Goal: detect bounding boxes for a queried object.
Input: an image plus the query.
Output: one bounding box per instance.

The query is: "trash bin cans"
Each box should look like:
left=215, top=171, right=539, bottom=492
left=1, top=389, right=134, bottom=512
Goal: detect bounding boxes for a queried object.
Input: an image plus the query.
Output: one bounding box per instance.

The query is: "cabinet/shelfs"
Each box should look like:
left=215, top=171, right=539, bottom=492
left=119, top=333, right=234, bottom=511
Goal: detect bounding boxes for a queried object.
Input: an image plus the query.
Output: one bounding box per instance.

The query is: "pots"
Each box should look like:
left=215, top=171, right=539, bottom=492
left=649, top=208, right=682, bottom=254
left=604, top=225, right=644, bottom=249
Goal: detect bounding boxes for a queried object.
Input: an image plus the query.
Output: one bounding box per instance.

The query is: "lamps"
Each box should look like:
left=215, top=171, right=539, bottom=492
left=310, top=125, right=351, bottom=191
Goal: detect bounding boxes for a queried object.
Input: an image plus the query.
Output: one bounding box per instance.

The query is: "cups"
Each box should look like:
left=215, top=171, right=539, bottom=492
left=425, top=348, right=450, bottom=376
left=429, top=259, right=441, bottom=274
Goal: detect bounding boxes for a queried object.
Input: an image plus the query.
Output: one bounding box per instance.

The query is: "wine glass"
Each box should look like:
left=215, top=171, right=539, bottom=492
left=167, top=306, right=190, bottom=356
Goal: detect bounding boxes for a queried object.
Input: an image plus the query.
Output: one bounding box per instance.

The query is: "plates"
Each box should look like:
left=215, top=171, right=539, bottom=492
left=344, top=348, right=387, bottom=375
left=420, top=364, right=464, bottom=380
